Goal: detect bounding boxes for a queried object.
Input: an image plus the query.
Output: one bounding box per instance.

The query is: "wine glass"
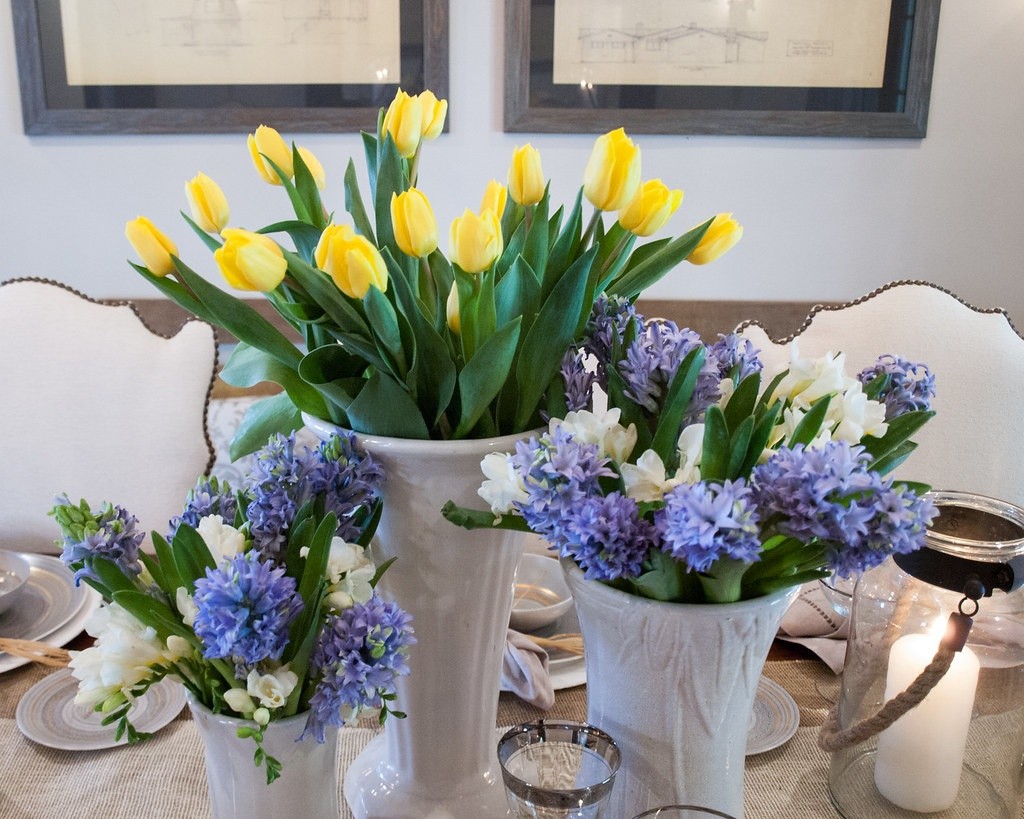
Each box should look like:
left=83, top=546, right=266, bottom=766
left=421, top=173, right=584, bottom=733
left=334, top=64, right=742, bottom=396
left=815, top=568, right=897, bottom=705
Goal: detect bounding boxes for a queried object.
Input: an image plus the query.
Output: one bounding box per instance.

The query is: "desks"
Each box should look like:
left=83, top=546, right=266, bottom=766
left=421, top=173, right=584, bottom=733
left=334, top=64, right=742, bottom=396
left=0, top=629, right=1024, bottom=819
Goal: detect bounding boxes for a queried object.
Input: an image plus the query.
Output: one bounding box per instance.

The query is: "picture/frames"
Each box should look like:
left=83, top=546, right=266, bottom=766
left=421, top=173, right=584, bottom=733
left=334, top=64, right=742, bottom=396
left=503, top=1, right=942, bottom=138
left=10, top=0, right=451, bottom=134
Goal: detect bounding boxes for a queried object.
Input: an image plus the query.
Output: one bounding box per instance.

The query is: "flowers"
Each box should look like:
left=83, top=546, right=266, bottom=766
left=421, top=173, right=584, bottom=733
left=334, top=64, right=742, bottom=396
left=44, top=86, right=941, bottom=790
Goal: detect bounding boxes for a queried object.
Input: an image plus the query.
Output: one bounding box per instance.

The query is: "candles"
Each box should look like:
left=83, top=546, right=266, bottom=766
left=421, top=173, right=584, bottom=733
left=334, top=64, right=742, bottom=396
left=873, top=633, right=980, bottom=813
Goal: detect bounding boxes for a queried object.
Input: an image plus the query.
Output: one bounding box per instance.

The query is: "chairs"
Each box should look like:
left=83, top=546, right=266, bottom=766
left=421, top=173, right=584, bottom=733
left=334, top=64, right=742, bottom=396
left=1, top=274, right=221, bottom=556
left=741, top=279, right=1023, bottom=504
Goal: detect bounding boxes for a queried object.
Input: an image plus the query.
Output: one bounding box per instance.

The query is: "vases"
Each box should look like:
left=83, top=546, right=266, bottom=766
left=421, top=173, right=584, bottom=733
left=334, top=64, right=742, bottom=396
left=496, top=552, right=803, bottom=819
left=298, top=410, right=549, bottom=819
left=185, top=686, right=340, bottom=819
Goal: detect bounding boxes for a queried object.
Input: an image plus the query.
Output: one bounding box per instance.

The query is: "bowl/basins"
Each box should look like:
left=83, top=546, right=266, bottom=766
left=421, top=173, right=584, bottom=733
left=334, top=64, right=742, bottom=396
left=510, top=553, right=574, bottom=631
left=0, top=551, right=31, bottom=614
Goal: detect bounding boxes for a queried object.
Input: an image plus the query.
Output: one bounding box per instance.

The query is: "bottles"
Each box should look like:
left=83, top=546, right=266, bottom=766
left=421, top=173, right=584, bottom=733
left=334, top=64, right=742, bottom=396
left=827, top=490, right=1024, bottom=819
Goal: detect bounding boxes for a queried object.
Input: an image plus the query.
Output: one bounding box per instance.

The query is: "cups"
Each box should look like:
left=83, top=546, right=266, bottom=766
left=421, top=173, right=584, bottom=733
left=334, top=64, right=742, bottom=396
left=497, top=722, right=622, bottom=819
left=634, top=805, right=736, bottom=819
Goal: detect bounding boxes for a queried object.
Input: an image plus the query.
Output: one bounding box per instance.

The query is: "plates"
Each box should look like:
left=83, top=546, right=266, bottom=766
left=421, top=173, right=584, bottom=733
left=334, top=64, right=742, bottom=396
left=548, top=608, right=583, bottom=670
left=0, top=586, right=102, bottom=673
left=16, top=669, right=187, bottom=750
left=499, top=659, right=587, bottom=693
left=0, top=553, right=88, bottom=654
left=745, top=675, right=799, bottom=757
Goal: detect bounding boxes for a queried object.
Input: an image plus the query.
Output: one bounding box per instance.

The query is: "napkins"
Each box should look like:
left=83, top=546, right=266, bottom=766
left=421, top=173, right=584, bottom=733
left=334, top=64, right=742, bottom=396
left=777, top=578, right=887, bottom=675
left=500, top=629, right=556, bottom=710
